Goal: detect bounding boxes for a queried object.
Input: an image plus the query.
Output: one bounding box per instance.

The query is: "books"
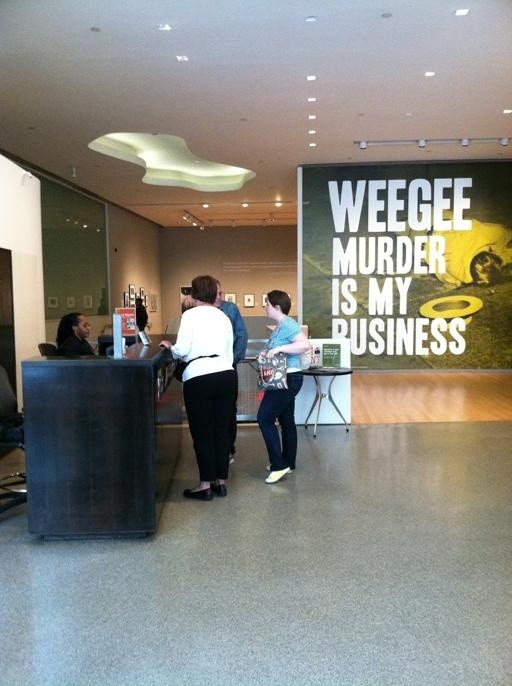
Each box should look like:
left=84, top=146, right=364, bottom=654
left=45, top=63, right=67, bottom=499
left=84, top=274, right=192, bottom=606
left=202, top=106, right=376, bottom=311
left=322, top=343, right=342, bottom=369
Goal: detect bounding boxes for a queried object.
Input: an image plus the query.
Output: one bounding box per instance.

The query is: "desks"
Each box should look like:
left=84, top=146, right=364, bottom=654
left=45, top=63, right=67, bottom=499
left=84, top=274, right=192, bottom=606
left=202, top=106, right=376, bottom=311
left=300, top=367, right=354, bottom=438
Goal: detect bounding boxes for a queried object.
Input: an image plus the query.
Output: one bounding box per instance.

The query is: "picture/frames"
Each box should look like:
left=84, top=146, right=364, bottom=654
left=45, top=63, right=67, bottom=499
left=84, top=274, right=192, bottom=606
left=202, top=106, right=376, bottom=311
left=179, top=285, right=197, bottom=319
left=46, top=296, right=92, bottom=311
left=123, top=284, right=159, bottom=312
left=224, top=293, right=290, bottom=308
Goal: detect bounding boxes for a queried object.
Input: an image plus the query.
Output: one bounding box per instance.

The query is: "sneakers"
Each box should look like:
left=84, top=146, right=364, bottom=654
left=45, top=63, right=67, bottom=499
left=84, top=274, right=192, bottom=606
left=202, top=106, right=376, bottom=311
left=264, top=466, right=291, bottom=484
left=266, top=463, right=292, bottom=474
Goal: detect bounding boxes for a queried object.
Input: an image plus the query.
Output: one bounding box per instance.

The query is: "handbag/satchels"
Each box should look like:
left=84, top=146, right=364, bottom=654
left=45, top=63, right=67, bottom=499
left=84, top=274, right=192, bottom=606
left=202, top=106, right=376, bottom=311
left=255, top=351, right=290, bottom=391
left=174, top=359, right=189, bottom=382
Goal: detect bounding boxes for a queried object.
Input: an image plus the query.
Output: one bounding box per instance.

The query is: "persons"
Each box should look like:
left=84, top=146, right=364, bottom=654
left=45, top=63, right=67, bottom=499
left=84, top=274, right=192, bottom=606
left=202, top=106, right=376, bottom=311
left=213, top=280, right=249, bottom=468
left=181, top=292, right=198, bottom=310
left=157, top=274, right=239, bottom=503
left=135, top=298, right=148, bottom=331
left=394, top=204, right=512, bottom=292
left=53, top=309, right=99, bottom=356
left=256, top=288, right=314, bottom=486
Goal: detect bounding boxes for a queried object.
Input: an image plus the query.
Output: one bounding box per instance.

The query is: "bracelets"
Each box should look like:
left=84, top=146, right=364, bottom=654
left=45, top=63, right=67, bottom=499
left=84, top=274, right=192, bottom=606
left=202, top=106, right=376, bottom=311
left=277, top=345, right=282, bottom=353
left=168, top=344, right=173, bottom=351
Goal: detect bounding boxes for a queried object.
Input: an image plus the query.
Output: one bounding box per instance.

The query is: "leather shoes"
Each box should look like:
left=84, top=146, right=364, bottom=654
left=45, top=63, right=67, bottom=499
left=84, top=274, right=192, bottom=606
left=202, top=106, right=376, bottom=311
left=183, top=486, right=213, bottom=501
left=210, top=483, right=227, bottom=497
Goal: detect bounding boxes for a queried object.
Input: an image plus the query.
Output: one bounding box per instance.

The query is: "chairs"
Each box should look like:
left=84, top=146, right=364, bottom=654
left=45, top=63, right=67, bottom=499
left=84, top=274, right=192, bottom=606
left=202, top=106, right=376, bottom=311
left=38, top=343, right=61, bottom=356
left=0, top=366, right=27, bottom=516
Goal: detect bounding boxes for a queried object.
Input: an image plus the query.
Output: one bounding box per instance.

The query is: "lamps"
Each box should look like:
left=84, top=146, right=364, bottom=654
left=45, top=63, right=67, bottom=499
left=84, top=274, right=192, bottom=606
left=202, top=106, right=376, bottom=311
left=352, top=136, right=512, bottom=151
left=181, top=209, right=207, bottom=232
left=58, top=210, right=104, bottom=232
